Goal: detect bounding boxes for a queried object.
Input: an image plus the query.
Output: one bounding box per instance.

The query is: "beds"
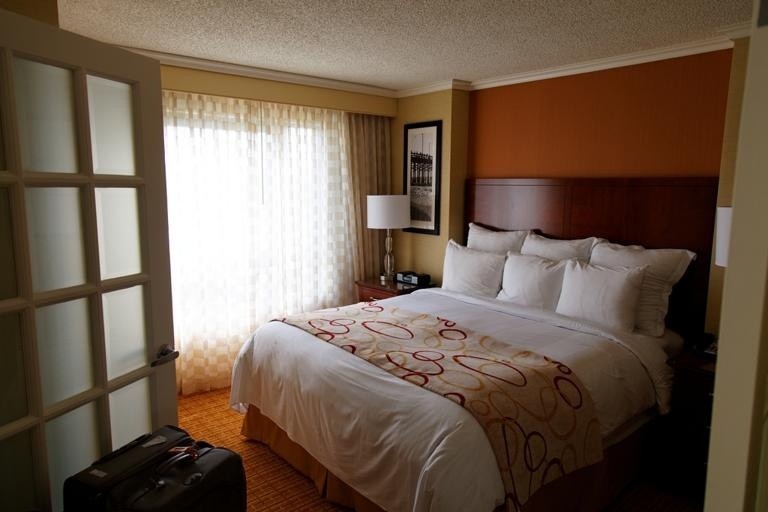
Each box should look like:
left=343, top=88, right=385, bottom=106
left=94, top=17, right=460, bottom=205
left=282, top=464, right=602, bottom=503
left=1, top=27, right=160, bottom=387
left=246, top=281, right=686, bottom=511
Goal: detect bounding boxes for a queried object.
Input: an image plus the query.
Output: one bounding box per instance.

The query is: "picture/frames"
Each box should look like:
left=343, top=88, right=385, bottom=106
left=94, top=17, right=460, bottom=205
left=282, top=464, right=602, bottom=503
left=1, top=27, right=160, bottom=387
left=400, top=119, right=440, bottom=235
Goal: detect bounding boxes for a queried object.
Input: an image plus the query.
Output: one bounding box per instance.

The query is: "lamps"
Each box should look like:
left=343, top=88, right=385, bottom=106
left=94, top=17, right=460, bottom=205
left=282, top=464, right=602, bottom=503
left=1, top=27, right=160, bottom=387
left=367, top=195, right=411, bottom=281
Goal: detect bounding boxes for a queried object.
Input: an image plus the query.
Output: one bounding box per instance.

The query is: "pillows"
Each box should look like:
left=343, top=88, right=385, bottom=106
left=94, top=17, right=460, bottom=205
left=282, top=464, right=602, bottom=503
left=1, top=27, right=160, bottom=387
left=439, top=223, right=696, bottom=337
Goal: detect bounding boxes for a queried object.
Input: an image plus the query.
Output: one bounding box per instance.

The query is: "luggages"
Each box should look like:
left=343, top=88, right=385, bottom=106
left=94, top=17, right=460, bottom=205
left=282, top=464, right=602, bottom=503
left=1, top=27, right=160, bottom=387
left=63, top=425, right=246, bottom=512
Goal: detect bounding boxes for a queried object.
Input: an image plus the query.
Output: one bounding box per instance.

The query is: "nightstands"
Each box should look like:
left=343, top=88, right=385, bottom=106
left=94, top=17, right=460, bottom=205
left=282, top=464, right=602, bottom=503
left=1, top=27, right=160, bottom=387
left=354, top=277, right=437, bottom=302
left=664, top=331, right=719, bottom=386
